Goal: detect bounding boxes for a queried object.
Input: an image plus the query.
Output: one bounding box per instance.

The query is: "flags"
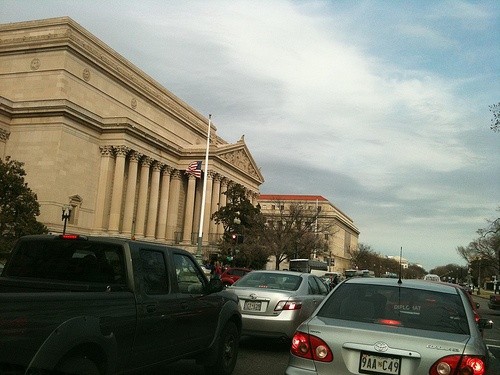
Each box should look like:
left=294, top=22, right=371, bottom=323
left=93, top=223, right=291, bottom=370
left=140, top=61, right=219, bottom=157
left=183, top=160, right=202, bottom=180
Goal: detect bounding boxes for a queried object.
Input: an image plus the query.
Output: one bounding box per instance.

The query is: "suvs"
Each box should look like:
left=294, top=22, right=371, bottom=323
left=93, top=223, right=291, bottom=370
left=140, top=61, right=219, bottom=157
left=220, top=269, right=249, bottom=286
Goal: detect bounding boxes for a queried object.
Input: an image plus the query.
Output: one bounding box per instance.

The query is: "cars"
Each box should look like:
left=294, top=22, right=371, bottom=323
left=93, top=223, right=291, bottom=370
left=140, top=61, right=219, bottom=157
left=286, top=278, right=493, bottom=375
left=226, top=271, right=329, bottom=337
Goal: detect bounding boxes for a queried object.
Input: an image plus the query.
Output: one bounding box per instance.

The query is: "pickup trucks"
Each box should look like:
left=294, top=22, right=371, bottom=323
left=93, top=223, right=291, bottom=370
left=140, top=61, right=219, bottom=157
left=0, top=234, right=244, bottom=375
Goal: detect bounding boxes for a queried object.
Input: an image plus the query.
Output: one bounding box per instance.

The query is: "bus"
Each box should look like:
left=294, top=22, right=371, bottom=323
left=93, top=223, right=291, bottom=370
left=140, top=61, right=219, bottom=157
left=285, top=258, right=329, bottom=277
